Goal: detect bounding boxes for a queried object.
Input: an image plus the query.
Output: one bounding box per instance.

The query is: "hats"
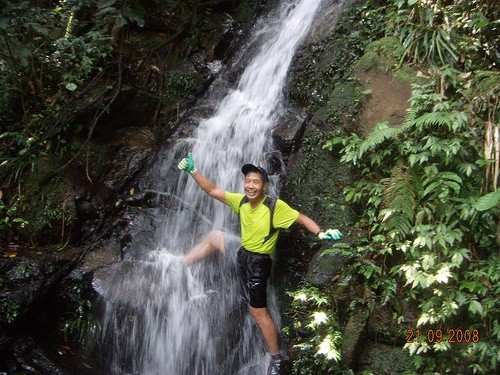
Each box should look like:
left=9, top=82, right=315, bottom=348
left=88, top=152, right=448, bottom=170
left=241, top=164, right=268, bottom=181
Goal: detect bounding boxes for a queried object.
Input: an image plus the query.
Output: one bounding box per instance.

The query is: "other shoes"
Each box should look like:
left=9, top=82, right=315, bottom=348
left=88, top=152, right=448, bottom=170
left=149, top=263, right=176, bottom=278
left=267, top=354, right=282, bottom=375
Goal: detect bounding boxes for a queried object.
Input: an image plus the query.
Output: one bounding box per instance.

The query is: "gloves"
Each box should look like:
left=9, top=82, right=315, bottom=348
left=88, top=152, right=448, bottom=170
left=317, top=229, right=343, bottom=240
left=177, top=152, right=198, bottom=176
